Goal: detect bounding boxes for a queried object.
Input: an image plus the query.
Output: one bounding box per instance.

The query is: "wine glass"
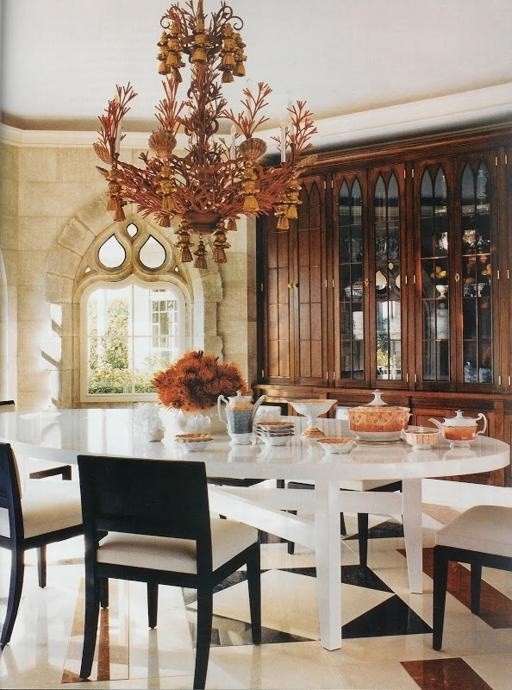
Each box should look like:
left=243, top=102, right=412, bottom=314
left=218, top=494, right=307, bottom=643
left=436, top=284, right=448, bottom=298
left=471, top=282, right=486, bottom=297
left=287, top=399, right=338, bottom=441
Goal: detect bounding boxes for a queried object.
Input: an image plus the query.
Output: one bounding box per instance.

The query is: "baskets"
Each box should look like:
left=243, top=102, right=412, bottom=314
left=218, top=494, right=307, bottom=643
left=349, top=407, right=410, bottom=431
left=402, top=428, right=439, bottom=446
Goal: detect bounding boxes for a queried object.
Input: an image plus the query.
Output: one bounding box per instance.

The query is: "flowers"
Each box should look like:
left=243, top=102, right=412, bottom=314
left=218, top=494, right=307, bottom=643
left=149, top=350, right=251, bottom=412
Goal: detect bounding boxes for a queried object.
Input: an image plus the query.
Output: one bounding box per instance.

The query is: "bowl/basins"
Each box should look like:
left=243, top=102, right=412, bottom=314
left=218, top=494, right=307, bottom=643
left=256, top=420, right=295, bottom=447
left=317, top=437, right=353, bottom=454
left=174, top=432, right=215, bottom=452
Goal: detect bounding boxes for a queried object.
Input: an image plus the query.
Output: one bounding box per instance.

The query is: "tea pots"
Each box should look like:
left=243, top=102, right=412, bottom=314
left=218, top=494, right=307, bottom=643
left=427, top=409, right=487, bottom=450
left=217, top=390, right=267, bottom=446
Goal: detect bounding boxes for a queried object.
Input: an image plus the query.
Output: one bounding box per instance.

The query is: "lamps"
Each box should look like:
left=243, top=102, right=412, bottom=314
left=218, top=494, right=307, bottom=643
left=90, top=0, right=319, bottom=268
